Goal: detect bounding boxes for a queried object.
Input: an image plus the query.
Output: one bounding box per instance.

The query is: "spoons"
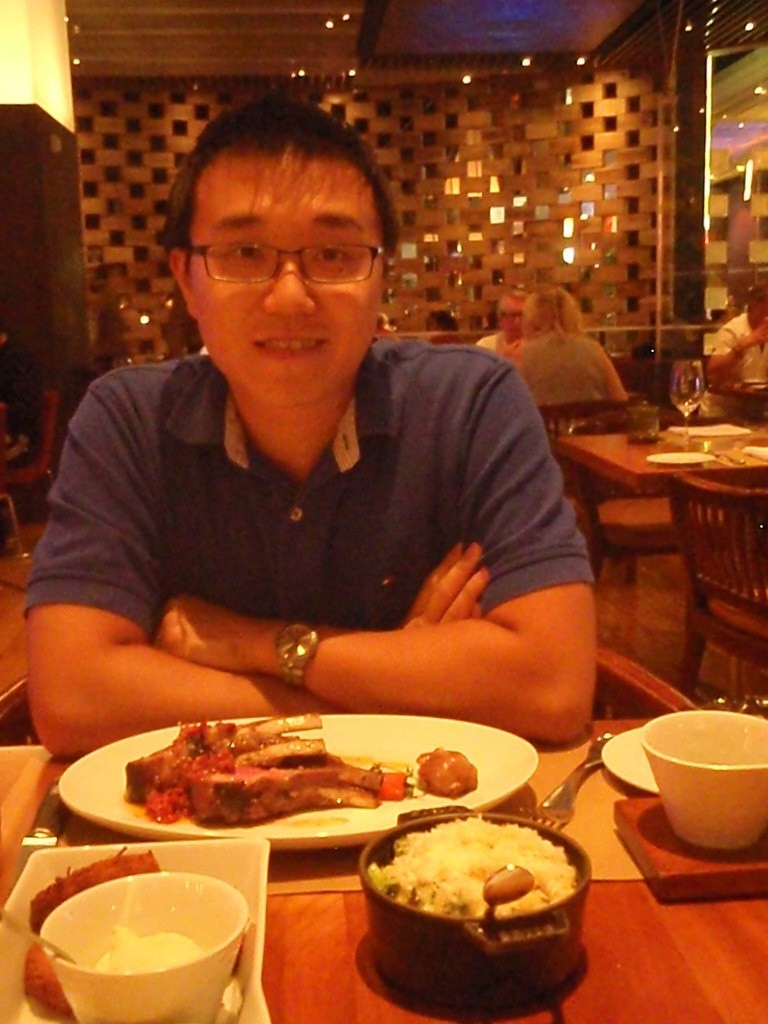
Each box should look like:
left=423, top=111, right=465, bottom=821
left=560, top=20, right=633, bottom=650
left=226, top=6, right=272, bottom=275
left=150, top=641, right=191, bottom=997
left=473, top=865, right=534, bottom=946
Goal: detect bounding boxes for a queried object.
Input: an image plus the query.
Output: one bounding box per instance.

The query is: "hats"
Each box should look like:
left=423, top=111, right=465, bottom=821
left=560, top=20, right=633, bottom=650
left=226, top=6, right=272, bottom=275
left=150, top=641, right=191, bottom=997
left=748, top=282, right=768, bottom=299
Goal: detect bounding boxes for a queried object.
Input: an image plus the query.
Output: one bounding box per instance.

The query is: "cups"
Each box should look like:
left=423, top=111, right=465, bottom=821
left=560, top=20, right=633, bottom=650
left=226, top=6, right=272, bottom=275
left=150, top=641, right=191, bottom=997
left=625, top=405, right=659, bottom=444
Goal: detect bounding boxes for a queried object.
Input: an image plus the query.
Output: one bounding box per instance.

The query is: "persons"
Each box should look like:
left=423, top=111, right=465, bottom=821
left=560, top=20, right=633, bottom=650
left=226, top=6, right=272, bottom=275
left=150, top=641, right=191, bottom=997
left=428, top=313, right=454, bottom=344
left=476, top=290, right=529, bottom=352
left=699, top=281, right=768, bottom=418
left=0, top=318, right=45, bottom=553
left=23, top=93, right=594, bottom=756
left=510, top=288, right=627, bottom=407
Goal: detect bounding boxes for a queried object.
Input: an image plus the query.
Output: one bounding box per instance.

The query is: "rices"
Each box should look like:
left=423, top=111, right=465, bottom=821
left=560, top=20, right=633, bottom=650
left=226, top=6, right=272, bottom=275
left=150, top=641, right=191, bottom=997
left=375, top=813, right=577, bottom=920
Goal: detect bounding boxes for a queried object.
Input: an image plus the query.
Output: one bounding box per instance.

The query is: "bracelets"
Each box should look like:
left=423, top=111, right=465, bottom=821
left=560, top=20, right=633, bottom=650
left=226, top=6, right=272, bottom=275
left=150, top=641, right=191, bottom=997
left=732, top=348, right=744, bottom=359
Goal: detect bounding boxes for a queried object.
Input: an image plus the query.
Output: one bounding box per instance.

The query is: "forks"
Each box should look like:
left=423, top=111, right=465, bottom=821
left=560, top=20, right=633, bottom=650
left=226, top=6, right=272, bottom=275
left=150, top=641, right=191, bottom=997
left=519, top=734, right=614, bottom=826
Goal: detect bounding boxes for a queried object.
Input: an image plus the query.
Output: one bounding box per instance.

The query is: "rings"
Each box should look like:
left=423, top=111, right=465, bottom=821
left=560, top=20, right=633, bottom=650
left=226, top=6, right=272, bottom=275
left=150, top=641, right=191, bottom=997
left=398, top=621, right=404, bottom=628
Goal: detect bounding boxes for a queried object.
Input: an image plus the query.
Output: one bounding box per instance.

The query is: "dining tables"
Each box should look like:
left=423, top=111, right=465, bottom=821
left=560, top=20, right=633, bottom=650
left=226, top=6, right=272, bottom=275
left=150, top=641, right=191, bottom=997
left=0, top=720, right=767, bottom=1024
left=557, top=426, right=767, bottom=490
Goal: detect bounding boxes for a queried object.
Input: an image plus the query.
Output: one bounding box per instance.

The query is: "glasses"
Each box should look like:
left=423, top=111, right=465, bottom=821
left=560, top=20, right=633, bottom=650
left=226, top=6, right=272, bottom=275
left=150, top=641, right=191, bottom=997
left=187, top=243, right=387, bottom=283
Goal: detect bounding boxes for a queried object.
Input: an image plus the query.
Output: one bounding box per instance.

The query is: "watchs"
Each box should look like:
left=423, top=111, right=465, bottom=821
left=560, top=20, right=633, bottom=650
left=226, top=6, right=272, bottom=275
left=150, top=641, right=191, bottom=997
left=276, top=624, right=317, bottom=685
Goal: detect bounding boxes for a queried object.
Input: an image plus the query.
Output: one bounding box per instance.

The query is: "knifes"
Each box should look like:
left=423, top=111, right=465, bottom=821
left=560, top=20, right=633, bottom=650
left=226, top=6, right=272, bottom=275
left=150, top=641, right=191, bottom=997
left=714, top=453, right=745, bottom=464
left=10, top=778, right=66, bottom=899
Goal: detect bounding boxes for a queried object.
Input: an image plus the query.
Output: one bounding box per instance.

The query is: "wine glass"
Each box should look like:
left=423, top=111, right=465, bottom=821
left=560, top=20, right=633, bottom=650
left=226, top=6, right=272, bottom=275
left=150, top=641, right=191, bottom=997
left=669, top=360, right=704, bottom=452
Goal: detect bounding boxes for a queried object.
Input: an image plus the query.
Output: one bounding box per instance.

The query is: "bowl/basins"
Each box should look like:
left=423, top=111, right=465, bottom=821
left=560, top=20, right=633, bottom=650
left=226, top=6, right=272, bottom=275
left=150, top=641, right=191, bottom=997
left=40, top=871, right=253, bottom=1023
left=641, top=710, right=768, bottom=849
left=358, top=805, right=591, bottom=1009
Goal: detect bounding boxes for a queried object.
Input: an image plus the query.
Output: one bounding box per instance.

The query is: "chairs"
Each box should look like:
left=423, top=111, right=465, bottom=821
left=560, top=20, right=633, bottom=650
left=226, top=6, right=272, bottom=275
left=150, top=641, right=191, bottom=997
left=537, top=400, right=635, bottom=448
left=0, top=403, right=22, bottom=556
left=666, top=472, right=767, bottom=710
left=6, top=386, right=60, bottom=484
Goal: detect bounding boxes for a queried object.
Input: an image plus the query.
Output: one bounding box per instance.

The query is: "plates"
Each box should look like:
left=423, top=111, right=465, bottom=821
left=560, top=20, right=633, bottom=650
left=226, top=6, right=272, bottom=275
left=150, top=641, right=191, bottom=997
left=1, top=837, right=274, bottom=1023
left=59, top=713, right=538, bottom=850
left=646, top=452, right=714, bottom=464
left=601, top=725, right=656, bottom=795
